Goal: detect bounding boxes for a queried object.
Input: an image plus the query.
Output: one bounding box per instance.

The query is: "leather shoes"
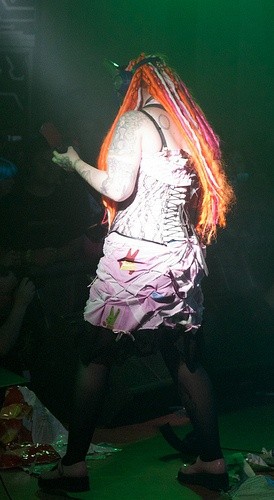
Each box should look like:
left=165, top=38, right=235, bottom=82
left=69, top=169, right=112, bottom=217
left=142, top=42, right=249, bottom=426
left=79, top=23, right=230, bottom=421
left=177, top=454, right=231, bottom=492
left=38, top=459, right=90, bottom=493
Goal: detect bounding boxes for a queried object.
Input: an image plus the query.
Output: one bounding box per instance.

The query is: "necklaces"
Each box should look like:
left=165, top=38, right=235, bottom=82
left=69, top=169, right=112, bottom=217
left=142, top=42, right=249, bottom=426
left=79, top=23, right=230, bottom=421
left=142, top=95, right=155, bottom=106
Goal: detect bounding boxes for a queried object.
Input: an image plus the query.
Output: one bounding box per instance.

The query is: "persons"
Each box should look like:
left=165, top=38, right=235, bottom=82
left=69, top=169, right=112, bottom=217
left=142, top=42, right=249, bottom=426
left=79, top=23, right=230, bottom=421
left=217, top=145, right=273, bottom=380
left=37, top=51, right=235, bottom=493
left=0, top=158, right=19, bottom=252
left=0, top=130, right=113, bottom=295
left=0, top=266, right=59, bottom=413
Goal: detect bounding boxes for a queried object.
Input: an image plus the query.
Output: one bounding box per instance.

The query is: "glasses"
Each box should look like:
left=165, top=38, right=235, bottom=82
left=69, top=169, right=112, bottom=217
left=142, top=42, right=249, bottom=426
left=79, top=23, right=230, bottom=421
left=111, top=71, right=133, bottom=97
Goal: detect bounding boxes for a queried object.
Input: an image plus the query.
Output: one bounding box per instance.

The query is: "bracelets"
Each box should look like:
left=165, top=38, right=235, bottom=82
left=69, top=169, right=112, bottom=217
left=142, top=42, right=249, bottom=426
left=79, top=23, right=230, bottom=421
left=24, top=249, right=33, bottom=273
